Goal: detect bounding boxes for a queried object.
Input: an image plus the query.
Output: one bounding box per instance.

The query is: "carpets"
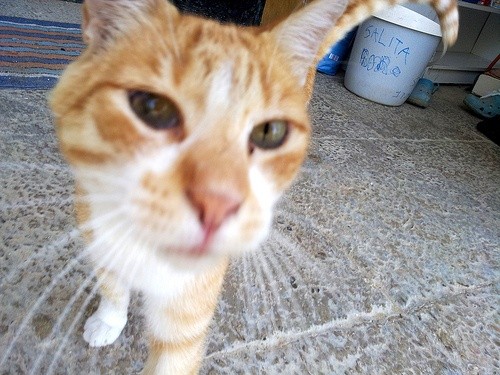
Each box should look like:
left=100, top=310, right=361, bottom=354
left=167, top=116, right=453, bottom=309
left=0, top=15, right=88, bottom=94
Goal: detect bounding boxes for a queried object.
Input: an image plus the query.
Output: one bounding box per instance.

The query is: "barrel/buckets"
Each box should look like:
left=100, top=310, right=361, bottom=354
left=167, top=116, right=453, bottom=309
left=343, top=4, right=442, bottom=107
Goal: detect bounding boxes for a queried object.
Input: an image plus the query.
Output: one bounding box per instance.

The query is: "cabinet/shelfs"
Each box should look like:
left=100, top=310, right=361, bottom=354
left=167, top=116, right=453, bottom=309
left=421, top=0, right=500, bottom=84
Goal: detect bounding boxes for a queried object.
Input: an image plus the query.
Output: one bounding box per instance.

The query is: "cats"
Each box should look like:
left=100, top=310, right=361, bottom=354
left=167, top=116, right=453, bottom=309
left=1, top=1, right=461, bottom=374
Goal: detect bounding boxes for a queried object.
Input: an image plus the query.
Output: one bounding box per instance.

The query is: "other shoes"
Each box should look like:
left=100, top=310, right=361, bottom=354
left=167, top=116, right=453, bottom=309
left=408, top=78, right=440, bottom=108
left=462, top=94, right=500, bottom=118
